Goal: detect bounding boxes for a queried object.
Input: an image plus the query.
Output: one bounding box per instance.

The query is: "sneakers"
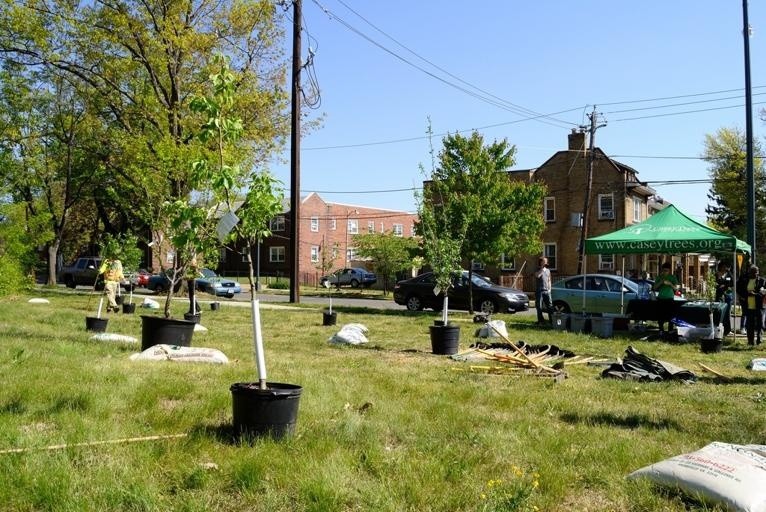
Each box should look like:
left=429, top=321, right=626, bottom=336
left=106, top=305, right=121, bottom=314
left=536, top=318, right=555, bottom=326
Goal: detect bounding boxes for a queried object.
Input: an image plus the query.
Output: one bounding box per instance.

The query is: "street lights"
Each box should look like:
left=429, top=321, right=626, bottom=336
left=345, top=209, right=359, bottom=268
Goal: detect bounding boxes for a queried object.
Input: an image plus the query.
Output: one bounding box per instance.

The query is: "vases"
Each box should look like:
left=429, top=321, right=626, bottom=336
left=552, top=313, right=569, bottom=332
left=570, top=313, right=590, bottom=333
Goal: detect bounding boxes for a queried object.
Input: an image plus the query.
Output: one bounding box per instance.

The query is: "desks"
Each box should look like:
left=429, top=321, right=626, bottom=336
left=627, top=298, right=728, bottom=336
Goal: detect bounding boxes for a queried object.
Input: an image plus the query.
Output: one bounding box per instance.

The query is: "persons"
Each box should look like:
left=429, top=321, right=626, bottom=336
left=98, top=253, right=128, bottom=312
left=624, top=259, right=766, bottom=347
left=534, top=257, right=553, bottom=326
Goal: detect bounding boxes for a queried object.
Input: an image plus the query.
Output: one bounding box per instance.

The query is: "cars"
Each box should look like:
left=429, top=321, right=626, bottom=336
left=394, top=270, right=687, bottom=317
left=319, top=268, right=377, bottom=287
left=61, top=258, right=240, bottom=298
